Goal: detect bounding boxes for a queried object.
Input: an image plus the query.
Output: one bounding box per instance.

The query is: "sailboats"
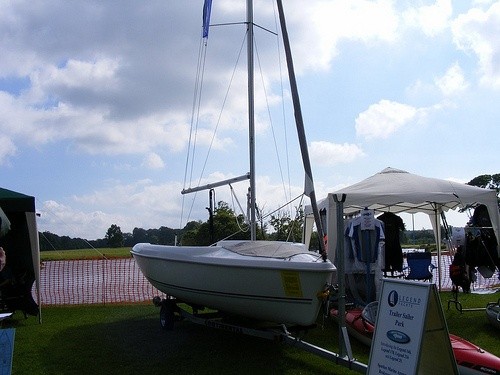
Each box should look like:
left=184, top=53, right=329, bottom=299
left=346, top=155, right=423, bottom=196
left=129, top=0, right=337, bottom=329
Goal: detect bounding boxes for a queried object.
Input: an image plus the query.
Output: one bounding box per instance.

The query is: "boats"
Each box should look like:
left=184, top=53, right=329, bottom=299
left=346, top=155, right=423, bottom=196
left=330, top=302, right=500, bottom=375
